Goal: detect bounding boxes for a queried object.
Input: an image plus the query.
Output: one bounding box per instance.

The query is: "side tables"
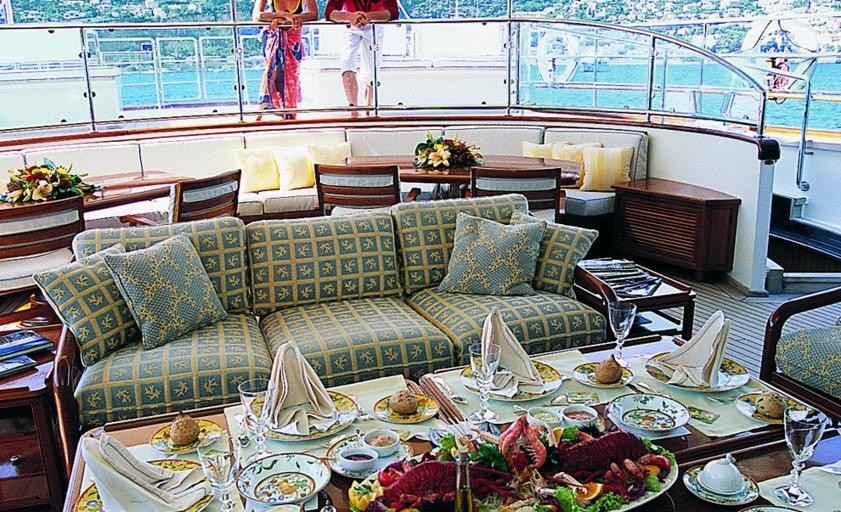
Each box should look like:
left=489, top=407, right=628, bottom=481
left=574, top=256, right=697, bottom=341
left=0, top=323, right=67, bottom=512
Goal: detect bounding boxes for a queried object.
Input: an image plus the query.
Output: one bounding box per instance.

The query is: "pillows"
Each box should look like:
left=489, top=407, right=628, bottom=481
left=551, top=143, right=603, bottom=188
left=437, top=213, right=545, bottom=295
left=580, top=146, right=633, bottom=192
left=309, top=142, right=352, bottom=169
left=34, top=243, right=142, bottom=367
left=509, top=210, right=598, bottom=299
left=238, top=146, right=279, bottom=192
left=523, top=140, right=552, bottom=159
left=104, top=236, right=229, bottom=350
left=272, top=146, right=315, bottom=191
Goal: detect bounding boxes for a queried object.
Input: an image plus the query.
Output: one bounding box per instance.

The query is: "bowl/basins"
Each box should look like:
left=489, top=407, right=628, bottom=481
left=704, top=457, right=745, bottom=494
left=564, top=405, right=599, bottom=427
left=258, top=505, right=303, bottom=512
left=608, top=394, right=691, bottom=431
left=364, top=429, right=399, bottom=457
left=339, top=447, right=378, bottom=473
left=527, top=405, right=561, bottom=430
left=235, top=451, right=332, bottom=505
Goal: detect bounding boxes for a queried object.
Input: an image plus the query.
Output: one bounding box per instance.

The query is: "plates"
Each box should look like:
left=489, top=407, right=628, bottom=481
left=350, top=428, right=680, bottom=511
left=249, top=386, right=360, bottom=443
left=327, top=432, right=413, bottom=481
left=685, top=461, right=761, bottom=506
left=459, top=360, right=563, bottom=401
left=571, top=360, right=634, bottom=388
left=374, top=393, right=440, bottom=422
left=644, top=350, right=752, bottom=393
left=150, top=419, right=222, bottom=455
left=74, top=460, right=219, bottom=511
left=737, top=506, right=803, bottom=512
left=735, top=392, right=807, bottom=428
left=697, top=470, right=747, bottom=496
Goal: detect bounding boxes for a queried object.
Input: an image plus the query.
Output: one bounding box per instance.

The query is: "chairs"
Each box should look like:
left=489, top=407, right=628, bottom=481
left=472, top=166, right=560, bottom=222
left=1, top=194, right=85, bottom=322
left=315, top=164, right=401, bottom=216
left=119, top=170, right=242, bottom=228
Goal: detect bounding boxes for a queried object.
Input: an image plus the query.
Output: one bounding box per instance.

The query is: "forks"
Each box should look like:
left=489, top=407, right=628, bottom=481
left=538, top=359, right=575, bottom=382
left=348, top=392, right=376, bottom=424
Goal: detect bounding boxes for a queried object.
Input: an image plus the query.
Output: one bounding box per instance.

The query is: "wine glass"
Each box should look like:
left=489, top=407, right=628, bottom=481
left=469, top=343, right=501, bottom=424
left=236, top=379, right=275, bottom=465
left=199, top=437, right=241, bottom=512
left=774, top=406, right=829, bottom=506
left=607, top=302, right=638, bottom=368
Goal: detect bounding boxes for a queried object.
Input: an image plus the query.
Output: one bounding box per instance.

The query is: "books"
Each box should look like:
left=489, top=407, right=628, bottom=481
left=0, top=331, right=55, bottom=362
left=1, top=355, right=37, bottom=380
left=578, top=256, right=661, bottom=298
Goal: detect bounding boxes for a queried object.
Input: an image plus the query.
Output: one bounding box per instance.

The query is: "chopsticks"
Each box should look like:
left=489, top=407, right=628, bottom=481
left=406, top=376, right=467, bottom=426
left=672, top=337, right=726, bottom=369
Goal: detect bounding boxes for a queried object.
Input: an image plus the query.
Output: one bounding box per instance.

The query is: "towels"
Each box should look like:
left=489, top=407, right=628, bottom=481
left=656, top=310, right=726, bottom=388
left=79, top=430, right=209, bottom=512
left=260, top=340, right=339, bottom=437
left=481, top=308, right=544, bottom=385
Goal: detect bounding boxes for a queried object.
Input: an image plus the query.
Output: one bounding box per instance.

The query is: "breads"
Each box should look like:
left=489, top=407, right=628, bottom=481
left=390, top=390, right=418, bottom=414
left=170, top=408, right=199, bottom=445
left=596, top=354, right=623, bottom=383
left=755, top=393, right=786, bottom=419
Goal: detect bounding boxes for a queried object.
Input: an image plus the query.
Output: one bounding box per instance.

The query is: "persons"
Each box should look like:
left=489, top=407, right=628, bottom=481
left=325, top=0, right=402, bottom=117
left=252, top=0, right=317, bottom=120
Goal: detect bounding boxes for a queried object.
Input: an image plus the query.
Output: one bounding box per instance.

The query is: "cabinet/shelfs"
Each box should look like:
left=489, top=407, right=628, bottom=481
left=610, top=178, right=741, bottom=282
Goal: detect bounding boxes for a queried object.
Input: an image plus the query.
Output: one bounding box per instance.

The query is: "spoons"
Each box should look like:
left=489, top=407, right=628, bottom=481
left=235, top=413, right=251, bottom=449
left=434, top=375, right=470, bottom=405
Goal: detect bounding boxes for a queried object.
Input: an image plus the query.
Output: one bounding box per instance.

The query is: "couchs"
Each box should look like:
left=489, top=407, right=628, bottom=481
left=0, top=127, right=650, bottom=291
left=54, top=196, right=608, bottom=485
left=761, top=285, right=840, bottom=425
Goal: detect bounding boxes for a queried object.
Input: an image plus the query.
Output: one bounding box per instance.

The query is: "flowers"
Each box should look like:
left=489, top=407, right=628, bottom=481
left=413, top=128, right=482, bottom=170
left=1, top=157, right=104, bottom=203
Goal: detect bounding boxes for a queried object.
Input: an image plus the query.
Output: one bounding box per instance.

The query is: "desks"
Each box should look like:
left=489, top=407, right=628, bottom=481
left=81, top=169, right=195, bottom=213
left=338, top=155, right=580, bottom=184
